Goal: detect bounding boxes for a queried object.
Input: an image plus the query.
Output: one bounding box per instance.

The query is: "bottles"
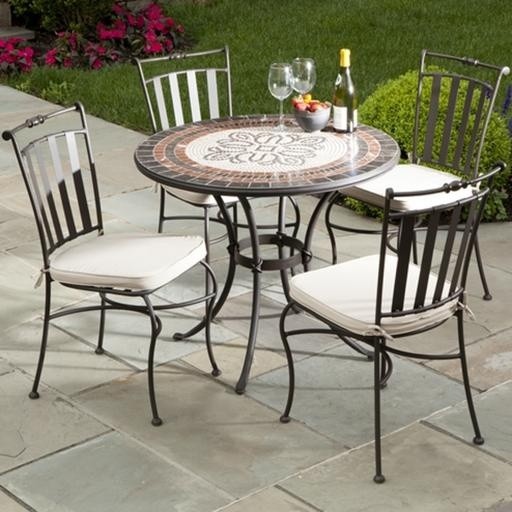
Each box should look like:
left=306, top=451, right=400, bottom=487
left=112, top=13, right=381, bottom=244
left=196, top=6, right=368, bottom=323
left=333, top=47, right=359, bottom=133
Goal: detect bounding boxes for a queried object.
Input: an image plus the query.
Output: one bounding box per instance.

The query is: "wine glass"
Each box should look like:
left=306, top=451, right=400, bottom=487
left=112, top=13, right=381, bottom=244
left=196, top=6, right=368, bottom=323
left=268, top=62, right=295, bottom=135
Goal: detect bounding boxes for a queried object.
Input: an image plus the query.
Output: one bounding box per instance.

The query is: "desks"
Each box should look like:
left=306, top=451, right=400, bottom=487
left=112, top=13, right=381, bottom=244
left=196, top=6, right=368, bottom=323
left=136, top=110, right=402, bottom=396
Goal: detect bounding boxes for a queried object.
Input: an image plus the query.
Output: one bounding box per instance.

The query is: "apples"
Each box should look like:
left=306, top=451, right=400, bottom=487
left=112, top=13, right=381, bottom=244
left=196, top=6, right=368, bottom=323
left=291, top=95, right=328, bottom=112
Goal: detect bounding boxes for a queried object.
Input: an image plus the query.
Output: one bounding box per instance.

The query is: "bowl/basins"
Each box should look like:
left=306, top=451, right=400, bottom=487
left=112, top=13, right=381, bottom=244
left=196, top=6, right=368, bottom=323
left=293, top=102, right=332, bottom=133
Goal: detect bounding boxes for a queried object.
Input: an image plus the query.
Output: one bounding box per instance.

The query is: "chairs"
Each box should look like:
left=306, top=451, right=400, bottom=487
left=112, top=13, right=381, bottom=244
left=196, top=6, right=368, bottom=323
left=322, top=47, right=511, bottom=301
left=279, top=162, right=506, bottom=485
left=132, top=43, right=301, bottom=259
left=0, top=100, right=221, bottom=426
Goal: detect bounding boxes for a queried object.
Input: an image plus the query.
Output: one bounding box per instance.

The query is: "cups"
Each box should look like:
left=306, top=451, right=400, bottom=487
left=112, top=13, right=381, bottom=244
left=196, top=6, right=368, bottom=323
left=290, top=57, right=317, bottom=94
